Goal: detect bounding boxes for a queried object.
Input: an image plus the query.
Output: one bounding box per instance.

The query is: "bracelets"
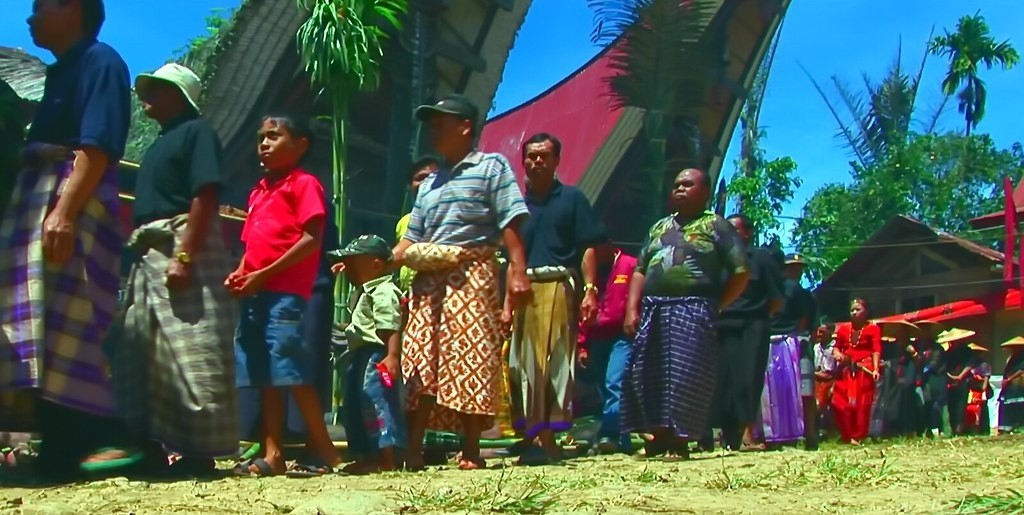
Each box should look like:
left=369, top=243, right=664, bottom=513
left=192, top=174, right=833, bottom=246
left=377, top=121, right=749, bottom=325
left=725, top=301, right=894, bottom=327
left=718, top=308, right=722, bottom=312
left=584, top=282, right=598, bottom=296
left=913, top=351, right=918, bottom=358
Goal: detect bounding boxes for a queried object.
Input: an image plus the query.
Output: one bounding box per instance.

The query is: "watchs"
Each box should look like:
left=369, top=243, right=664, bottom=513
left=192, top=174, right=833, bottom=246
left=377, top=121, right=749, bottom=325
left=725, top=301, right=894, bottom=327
left=176, top=251, right=195, bottom=266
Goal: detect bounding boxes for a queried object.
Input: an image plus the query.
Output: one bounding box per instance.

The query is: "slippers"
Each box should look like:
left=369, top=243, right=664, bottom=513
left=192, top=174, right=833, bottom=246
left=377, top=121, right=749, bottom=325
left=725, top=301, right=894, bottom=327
left=79, top=443, right=144, bottom=471
left=233, top=457, right=275, bottom=478
left=285, top=461, right=334, bottom=478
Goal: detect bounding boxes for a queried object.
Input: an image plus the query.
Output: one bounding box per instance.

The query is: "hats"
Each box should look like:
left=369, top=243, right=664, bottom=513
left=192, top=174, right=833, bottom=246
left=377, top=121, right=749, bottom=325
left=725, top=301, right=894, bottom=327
left=416, top=92, right=479, bottom=130
left=784, top=253, right=808, bottom=266
left=875, top=318, right=990, bottom=352
left=1000, top=336, right=1024, bottom=348
left=135, top=62, right=203, bottom=114
left=326, top=233, right=393, bottom=265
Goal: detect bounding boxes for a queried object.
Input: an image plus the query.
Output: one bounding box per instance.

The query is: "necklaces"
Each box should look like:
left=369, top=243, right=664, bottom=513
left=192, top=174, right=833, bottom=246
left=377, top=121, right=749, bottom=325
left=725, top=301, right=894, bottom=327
left=849, top=321, right=866, bottom=348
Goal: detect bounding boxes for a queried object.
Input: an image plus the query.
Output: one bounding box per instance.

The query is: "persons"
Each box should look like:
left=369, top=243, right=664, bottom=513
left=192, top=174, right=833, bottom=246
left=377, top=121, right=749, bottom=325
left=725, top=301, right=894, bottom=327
left=394, top=155, right=441, bottom=293
left=499, top=131, right=600, bottom=461
left=622, top=167, right=750, bottom=464
left=448, top=307, right=515, bottom=466
left=0, top=1, right=131, bottom=474
left=222, top=112, right=344, bottom=477
left=689, top=213, right=788, bottom=457
left=578, top=224, right=640, bottom=453
left=76, top=63, right=241, bottom=479
left=331, top=92, right=533, bottom=469
left=782, top=253, right=1024, bottom=446
left=339, top=235, right=409, bottom=472
left=741, top=243, right=811, bottom=450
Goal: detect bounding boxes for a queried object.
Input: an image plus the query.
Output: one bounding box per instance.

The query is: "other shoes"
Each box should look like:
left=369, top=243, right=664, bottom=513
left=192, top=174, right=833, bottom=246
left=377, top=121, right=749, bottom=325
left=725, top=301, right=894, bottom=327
left=599, top=432, right=622, bottom=454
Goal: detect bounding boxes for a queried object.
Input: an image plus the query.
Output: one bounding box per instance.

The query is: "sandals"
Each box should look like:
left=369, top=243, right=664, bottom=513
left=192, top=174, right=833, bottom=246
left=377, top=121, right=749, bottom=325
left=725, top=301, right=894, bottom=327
left=632, top=444, right=690, bottom=461
